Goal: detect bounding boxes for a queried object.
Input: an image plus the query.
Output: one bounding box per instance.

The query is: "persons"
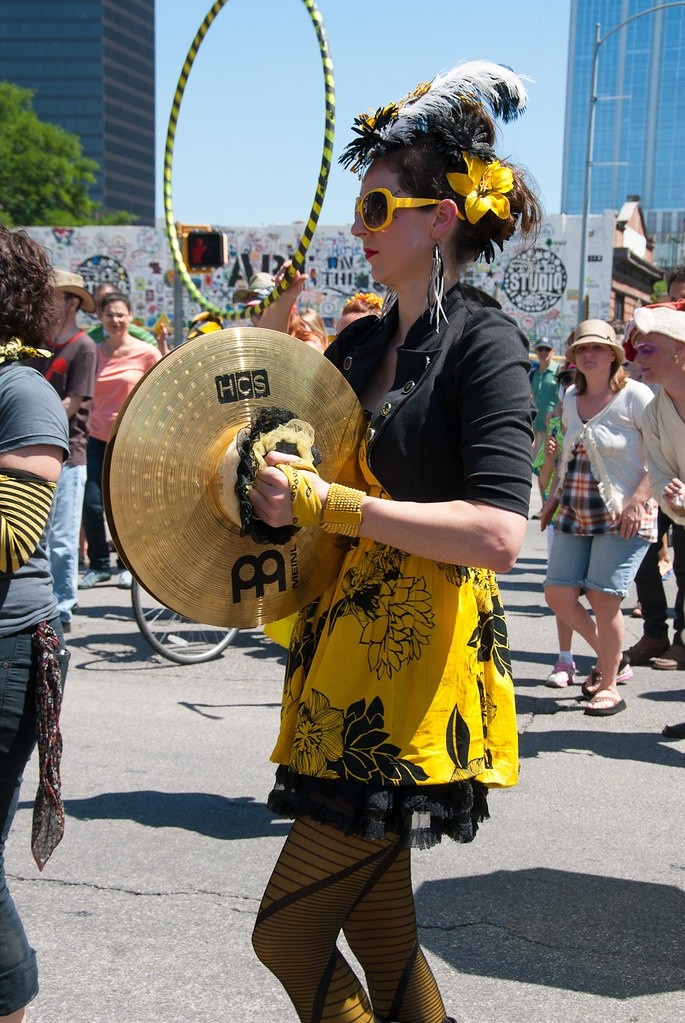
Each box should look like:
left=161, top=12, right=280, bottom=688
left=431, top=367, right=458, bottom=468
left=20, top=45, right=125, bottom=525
left=251, top=59, right=530, bottom=1022
left=240, top=267, right=685, bottom=738
left=0, top=227, right=222, bottom=1022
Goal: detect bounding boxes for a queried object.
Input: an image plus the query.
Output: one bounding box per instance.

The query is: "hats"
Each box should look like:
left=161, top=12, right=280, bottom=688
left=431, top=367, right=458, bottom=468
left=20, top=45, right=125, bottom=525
left=46, top=266, right=96, bottom=314
left=565, top=319, right=624, bottom=366
left=232, top=272, right=276, bottom=303
left=535, top=337, right=553, bottom=350
left=622, top=298, right=685, bottom=362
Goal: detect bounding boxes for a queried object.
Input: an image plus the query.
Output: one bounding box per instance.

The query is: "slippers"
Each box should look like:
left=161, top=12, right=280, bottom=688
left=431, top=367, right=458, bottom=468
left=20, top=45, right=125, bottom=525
left=583, top=696, right=627, bottom=717
left=582, top=654, right=630, bottom=696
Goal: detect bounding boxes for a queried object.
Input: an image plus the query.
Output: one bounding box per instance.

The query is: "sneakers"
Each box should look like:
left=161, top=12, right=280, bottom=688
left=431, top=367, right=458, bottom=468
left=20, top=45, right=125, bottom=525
left=622, top=636, right=670, bottom=665
left=78, top=568, right=111, bottom=589
left=652, top=643, right=685, bottom=670
left=545, top=660, right=576, bottom=688
left=118, top=569, right=133, bottom=589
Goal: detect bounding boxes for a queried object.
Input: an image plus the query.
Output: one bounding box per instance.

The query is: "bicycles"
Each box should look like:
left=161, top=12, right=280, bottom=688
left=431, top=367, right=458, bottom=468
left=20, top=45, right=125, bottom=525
left=130, top=574, right=241, bottom=665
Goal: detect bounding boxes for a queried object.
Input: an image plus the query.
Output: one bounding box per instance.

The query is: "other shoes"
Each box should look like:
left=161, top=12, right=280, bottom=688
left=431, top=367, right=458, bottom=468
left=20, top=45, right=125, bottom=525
left=662, top=722, right=685, bottom=739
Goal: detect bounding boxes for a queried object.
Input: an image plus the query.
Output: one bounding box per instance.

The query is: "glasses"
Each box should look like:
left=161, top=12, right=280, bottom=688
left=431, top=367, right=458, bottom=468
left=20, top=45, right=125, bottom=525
left=539, top=346, right=551, bottom=352
left=102, top=311, right=129, bottom=319
left=355, top=188, right=466, bottom=232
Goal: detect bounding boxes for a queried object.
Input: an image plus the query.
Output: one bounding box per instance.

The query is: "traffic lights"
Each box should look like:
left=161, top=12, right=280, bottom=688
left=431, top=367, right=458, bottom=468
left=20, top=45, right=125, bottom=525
left=182, top=228, right=228, bottom=272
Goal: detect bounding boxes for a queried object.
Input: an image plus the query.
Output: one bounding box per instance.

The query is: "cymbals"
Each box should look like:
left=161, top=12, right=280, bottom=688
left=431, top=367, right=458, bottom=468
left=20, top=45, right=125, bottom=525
left=98, top=327, right=366, bottom=630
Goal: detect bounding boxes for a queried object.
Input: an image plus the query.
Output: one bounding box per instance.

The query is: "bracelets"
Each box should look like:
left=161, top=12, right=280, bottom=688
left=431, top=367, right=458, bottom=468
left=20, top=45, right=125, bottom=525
left=321, top=483, right=365, bottom=538
